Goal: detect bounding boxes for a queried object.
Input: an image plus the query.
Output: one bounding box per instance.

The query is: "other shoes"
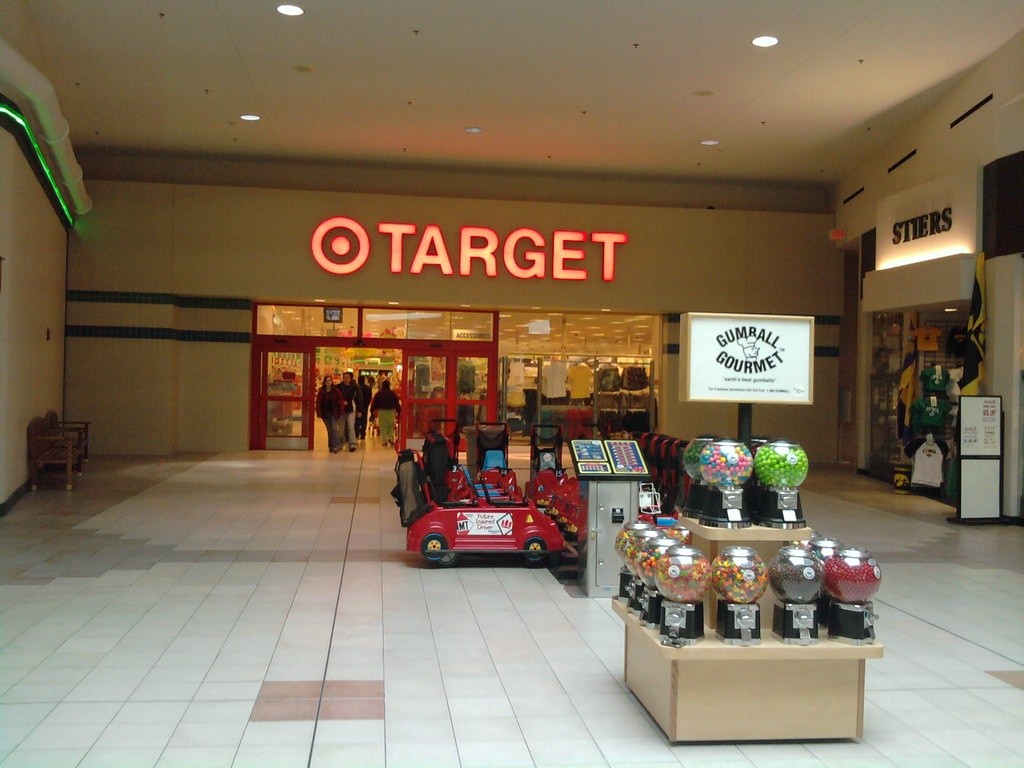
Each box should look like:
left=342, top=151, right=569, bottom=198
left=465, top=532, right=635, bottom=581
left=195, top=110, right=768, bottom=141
left=391, top=442, right=394, bottom=445
left=360, top=437, right=365, bottom=440
left=333, top=449, right=338, bottom=454
left=382, top=442, right=387, bottom=446
left=356, top=433, right=359, bottom=438
left=350, top=443, right=356, bottom=452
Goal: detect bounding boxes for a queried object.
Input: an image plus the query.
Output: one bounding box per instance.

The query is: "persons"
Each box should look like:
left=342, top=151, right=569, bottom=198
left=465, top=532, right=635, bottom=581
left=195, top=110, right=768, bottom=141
left=368, top=380, right=401, bottom=447
left=316, top=376, right=345, bottom=454
left=337, top=372, right=372, bottom=452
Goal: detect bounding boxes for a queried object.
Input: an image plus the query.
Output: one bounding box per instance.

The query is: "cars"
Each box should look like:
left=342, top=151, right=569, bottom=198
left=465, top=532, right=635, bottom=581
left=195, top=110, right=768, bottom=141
left=394, top=416, right=581, bottom=566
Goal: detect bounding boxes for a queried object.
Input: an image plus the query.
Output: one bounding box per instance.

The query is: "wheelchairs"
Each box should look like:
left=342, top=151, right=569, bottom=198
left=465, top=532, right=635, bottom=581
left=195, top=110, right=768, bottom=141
left=368, top=409, right=399, bottom=437
